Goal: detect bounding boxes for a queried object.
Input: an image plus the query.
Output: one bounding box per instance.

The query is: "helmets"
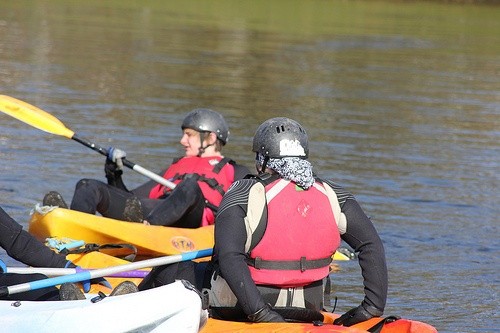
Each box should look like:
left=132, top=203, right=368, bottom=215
left=252, top=116, right=309, bottom=157
left=181, top=108, right=229, bottom=145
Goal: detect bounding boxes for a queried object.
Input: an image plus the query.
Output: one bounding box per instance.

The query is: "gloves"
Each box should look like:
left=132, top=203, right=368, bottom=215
left=248, top=301, right=286, bottom=323
left=332, top=305, right=374, bottom=326
left=104, top=146, right=127, bottom=177
left=65, top=260, right=112, bottom=293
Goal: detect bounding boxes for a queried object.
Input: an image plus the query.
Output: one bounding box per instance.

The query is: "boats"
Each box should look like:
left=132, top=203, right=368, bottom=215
left=45, top=238, right=438, bottom=333
left=0, top=279, right=208, bottom=333
left=28, top=201, right=358, bottom=260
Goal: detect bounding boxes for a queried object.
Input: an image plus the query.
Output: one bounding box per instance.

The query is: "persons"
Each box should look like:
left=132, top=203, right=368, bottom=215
left=43, top=108, right=251, bottom=229
left=0, top=207, right=137, bottom=302
left=139, top=117, right=388, bottom=327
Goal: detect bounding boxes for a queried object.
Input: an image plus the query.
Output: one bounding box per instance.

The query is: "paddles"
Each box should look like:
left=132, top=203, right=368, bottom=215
left=0, top=245, right=215, bottom=300
left=0, top=94, right=177, bottom=191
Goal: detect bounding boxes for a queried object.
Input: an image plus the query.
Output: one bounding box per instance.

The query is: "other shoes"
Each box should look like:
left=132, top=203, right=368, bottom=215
left=59, top=282, right=86, bottom=300
left=109, top=280, right=139, bottom=296
left=43, top=190, right=68, bottom=208
left=122, top=195, right=144, bottom=223
